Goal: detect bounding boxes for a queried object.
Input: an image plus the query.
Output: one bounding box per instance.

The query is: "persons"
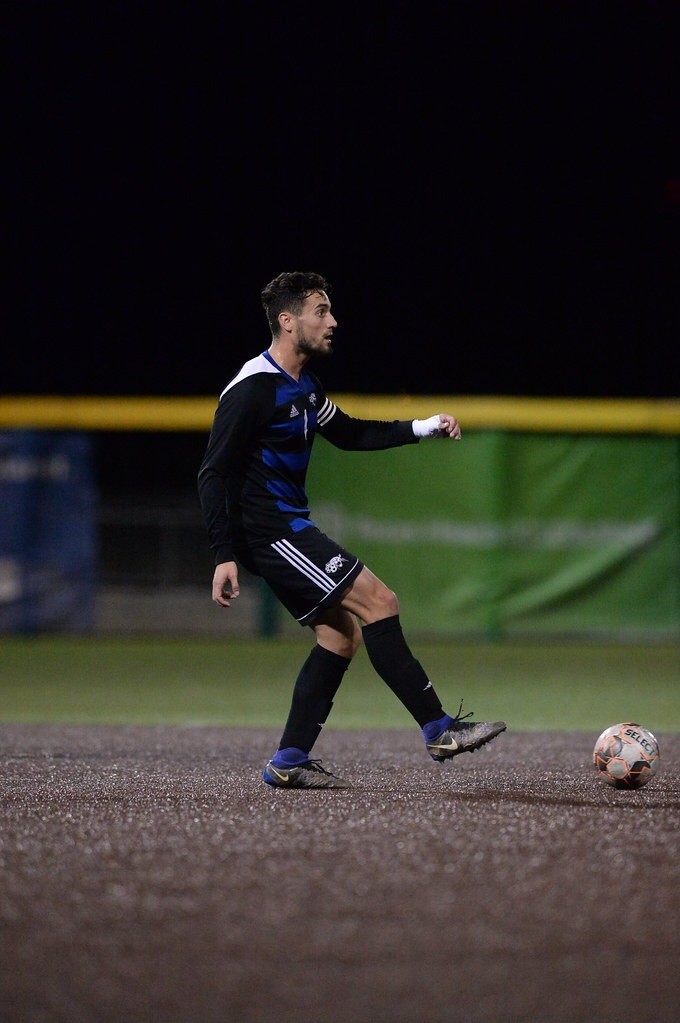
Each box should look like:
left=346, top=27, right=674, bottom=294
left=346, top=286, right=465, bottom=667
left=195, top=272, right=508, bottom=788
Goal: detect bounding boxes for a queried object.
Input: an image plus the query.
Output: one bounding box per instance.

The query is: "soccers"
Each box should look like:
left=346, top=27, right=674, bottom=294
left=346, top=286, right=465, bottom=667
left=592, top=721, right=659, bottom=790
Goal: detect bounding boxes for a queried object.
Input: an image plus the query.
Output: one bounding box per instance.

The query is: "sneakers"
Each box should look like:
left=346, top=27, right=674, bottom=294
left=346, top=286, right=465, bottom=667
left=423, top=698, right=507, bottom=763
left=263, top=748, right=352, bottom=789
